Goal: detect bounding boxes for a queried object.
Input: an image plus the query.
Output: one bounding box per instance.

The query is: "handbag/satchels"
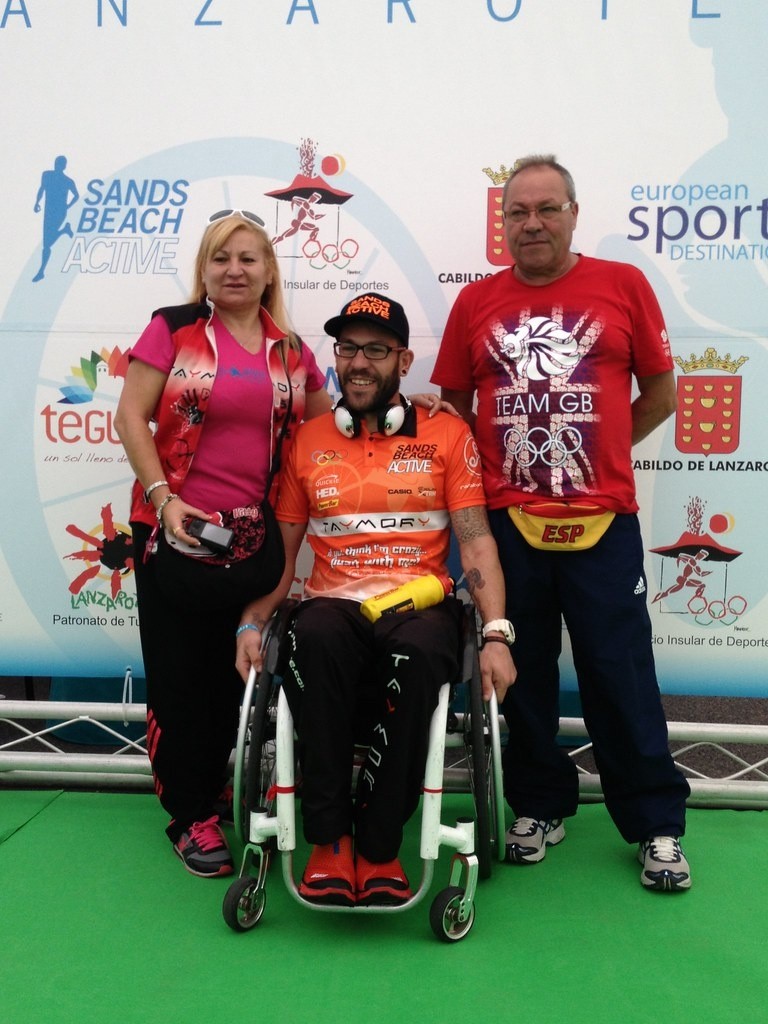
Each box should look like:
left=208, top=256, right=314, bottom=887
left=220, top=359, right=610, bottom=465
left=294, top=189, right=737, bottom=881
left=163, top=502, right=271, bottom=589
left=508, top=500, right=616, bottom=551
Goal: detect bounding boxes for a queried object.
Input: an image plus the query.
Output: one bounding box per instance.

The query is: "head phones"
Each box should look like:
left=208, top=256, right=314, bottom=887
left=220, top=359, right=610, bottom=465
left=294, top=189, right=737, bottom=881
left=331, top=389, right=412, bottom=438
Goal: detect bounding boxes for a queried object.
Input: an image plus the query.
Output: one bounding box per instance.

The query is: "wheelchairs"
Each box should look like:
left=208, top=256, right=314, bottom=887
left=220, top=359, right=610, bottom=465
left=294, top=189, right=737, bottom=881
left=221, top=595, right=508, bottom=944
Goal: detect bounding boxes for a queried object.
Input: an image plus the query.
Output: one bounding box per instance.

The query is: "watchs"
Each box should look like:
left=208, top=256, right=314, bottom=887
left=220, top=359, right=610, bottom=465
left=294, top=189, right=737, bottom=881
left=142, top=481, right=169, bottom=505
left=482, top=618, right=515, bottom=646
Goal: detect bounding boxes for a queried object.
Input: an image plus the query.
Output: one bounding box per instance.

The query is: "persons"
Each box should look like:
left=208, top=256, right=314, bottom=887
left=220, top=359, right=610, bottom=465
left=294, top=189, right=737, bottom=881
left=233, top=292, right=518, bottom=907
left=431, top=155, right=691, bottom=892
left=113, top=207, right=466, bottom=878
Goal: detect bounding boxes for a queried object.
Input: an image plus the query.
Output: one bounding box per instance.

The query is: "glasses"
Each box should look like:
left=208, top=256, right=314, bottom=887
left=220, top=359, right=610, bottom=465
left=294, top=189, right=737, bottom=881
left=503, top=201, right=571, bottom=221
left=333, top=342, right=406, bottom=360
left=206, top=208, right=267, bottom=232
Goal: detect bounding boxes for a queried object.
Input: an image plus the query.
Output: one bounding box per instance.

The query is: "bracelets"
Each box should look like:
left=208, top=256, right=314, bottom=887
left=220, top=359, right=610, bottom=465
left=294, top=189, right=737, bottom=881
left=156, top=493, right=181, bottom=529
left=484, top=636, right=510, bottom=646
left=236, top=624, right=258, bottom=638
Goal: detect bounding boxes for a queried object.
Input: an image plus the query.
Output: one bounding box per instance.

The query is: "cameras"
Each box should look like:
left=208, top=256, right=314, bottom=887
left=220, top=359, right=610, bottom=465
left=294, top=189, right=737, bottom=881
left=188, top=519, right=233, bottom=552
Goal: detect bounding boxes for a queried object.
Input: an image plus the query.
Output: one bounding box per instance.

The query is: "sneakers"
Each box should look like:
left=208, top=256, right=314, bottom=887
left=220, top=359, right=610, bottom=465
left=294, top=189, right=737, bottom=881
left=299, top=834, right=355, bottom=907
left=173, top=816, right=235, bottom=877
left=217, top=788, right=247, bottom=825
left=504, top=817, right=566, bottom=864
left=355, top=849, right=412, bottom=906
left=637, top=836, right=691, bottom=891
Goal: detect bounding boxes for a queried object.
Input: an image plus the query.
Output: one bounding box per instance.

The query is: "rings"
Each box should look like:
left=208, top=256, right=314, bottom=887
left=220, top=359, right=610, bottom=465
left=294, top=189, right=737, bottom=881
left=172, top=526, right=183, bottom=535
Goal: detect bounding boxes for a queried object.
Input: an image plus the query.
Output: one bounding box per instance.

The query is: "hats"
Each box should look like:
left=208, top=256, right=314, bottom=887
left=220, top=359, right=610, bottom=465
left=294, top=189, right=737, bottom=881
left=324, top=293, right=409, bottom=347
